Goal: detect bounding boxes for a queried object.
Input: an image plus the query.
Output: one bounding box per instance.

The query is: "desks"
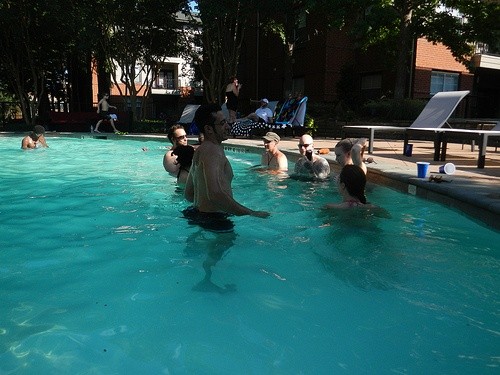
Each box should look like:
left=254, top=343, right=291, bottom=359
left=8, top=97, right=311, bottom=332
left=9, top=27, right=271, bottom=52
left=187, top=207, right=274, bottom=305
left=462, top=119, right=499, bottom=152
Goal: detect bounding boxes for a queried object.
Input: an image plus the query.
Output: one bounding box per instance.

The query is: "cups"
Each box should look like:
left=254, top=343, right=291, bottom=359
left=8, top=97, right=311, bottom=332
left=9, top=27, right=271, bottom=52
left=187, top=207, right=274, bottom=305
left=439, top=163, right=456, bottom=175
left=416, top=162, right=430, bottom=178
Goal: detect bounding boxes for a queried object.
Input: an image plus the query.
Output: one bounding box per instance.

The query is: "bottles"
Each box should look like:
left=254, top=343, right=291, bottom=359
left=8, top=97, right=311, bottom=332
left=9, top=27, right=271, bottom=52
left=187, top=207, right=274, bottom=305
left=317, top=148, right=329, bottom=153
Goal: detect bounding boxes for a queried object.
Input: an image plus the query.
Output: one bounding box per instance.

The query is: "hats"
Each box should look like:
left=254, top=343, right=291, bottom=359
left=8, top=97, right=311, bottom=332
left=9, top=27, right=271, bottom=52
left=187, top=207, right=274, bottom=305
left=261, top=132, right=280, bottom=142
left=259, top=98, right=268, bottom=103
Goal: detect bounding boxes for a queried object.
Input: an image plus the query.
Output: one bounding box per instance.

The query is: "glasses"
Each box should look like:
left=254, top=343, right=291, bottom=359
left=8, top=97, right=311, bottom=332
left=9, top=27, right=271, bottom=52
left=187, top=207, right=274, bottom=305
left=176, top=135, right=186, bottom=139
left=298, top=144, right=310, bottom=148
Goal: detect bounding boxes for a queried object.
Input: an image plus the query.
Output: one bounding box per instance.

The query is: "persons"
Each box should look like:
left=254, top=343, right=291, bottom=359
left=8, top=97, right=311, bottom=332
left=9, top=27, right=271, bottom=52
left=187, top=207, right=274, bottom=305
left=163, top=76, right=393, bottom=238
left=21, top=125, right=47, bottom=149
left=94, top=92, right=118, bottom=134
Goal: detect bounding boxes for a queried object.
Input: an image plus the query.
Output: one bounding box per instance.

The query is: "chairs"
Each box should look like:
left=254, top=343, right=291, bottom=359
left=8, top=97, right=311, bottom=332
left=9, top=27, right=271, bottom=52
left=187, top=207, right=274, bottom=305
left=165, top=104, right=201, bottom=134
left=228, top=101, right=279, bottom=126
left=342, top=90, right=470, bottom=154
left=230, top=96, right=308, bottom=139
left=405, top=122, right=500, bottom=169
